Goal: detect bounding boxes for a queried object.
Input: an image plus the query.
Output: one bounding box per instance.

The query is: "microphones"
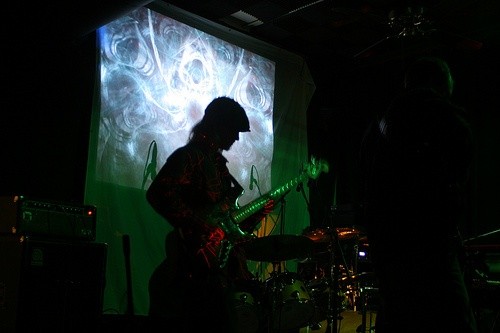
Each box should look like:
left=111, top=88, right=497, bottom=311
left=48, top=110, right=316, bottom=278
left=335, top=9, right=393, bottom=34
left=151, top=142, right=158, bottom=180
left=249, top=166, right=253, bottom=191
left=296, top=182, right=302, bottom=192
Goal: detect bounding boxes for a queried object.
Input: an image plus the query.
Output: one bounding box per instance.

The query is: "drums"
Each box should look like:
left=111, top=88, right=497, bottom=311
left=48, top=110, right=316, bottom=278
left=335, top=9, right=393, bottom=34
left=305, top=276, right=343, bottom=323
left=271, top=277, right=316, bottom=331
left=228, top=291, right=260, bottom=333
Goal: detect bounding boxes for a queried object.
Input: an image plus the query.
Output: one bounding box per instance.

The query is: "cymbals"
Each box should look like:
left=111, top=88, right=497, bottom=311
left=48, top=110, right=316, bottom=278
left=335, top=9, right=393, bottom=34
left=231, top=235, right=320, bottom=262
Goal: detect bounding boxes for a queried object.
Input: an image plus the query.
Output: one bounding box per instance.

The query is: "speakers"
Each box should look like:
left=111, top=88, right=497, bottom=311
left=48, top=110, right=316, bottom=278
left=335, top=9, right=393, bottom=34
left=0, top=231, right=107, bottom=333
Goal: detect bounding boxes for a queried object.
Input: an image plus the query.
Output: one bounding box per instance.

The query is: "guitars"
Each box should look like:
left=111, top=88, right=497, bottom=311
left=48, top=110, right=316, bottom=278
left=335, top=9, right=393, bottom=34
left=178, top=158, right=331, bottom=275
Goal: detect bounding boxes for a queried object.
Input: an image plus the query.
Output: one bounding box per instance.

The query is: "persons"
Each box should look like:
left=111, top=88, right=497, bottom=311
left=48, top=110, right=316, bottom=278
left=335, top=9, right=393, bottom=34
left=357, top=56, right=476, bottom=333
left=146, top=97, right=274, bottom=282
